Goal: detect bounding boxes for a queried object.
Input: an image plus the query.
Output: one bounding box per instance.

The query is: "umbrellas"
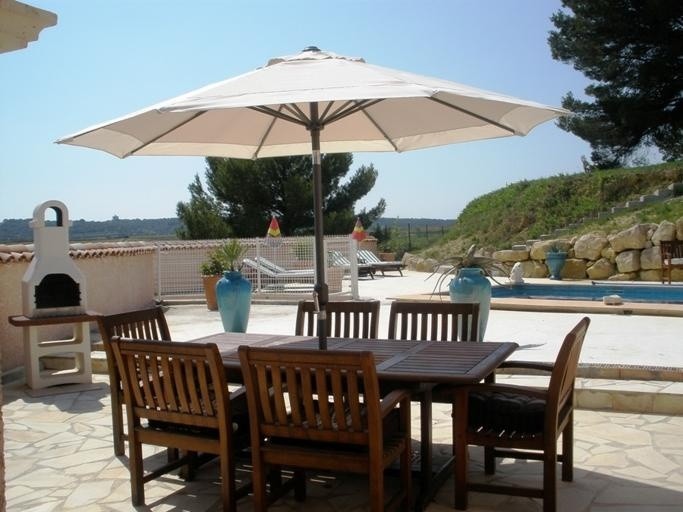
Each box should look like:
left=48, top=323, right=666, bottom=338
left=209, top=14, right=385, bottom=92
left=351, top=217, right=368, bottom=264
left=265, top=215, right=282, bottom=248
left=53, top=46, right=576, bottom=350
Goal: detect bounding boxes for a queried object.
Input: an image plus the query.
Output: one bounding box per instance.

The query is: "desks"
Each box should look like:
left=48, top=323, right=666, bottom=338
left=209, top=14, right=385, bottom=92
left=148, top=329, right=520, bottom=512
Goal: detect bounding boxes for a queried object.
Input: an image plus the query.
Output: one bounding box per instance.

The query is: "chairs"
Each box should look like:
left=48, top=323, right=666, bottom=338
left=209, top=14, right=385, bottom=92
left=96, top=305, right=192, bottom=467
left=290, top=302, right=382, bottom=342
left=106, top=335, right=246, bottom=509
left=660, top=238, right=683, bottom=285
left=454, top=315, right=591, bottom=510
left=387, top=300, right=482, bottom=343
left=237, top=345, right=413, bottom=511
left=243, top=247, right=405, bottom=283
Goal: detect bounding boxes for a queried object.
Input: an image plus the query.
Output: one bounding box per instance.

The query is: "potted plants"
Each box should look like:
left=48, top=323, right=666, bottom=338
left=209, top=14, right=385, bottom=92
left=200, top=236, right=251, bottom=332
left=425, top=242, right=511, bottom=344
left=543, top=241, right=568, bottom=280
